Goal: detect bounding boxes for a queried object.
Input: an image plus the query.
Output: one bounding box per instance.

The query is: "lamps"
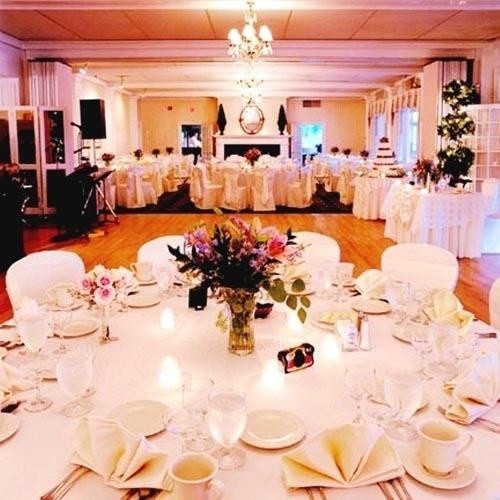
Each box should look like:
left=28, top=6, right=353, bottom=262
left=227, top=0, right=274, bottom=62
left=236, top=65, right=264, bottom=107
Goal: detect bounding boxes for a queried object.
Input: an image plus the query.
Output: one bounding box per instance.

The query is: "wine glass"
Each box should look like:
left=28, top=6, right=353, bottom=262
left=181, top=377, right=214, bottom=440
left=208, top=387, right=247, bottom=471
left=47, top=301, right=73, bottom=355
left=16, top=349, right=53, bottom=413
left=411, top=326, right=436, bottom=381
left=160, top=392, right=203, bottom=453
left=453, top=333, right=481, bottom=377
left=56, top=353, right=94, bottom=418
left=330, top=262, right=354, bottom=302
left=344, top=365, right=377, bottom=424
left=383, top=367, right=424, bottom=442
left=66, top=340, right=96, bottom=398
left=392, top=281, right=413, bottom=325
left=14, top=309, right=48, bottom=355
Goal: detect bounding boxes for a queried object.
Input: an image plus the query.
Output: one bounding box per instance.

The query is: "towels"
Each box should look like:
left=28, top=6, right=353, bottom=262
left=443, top=363, right=500, bottom=424
left=281, top=422, right=405, bottom=490
left=420, top=290, right=474, bottom=338
left=69, top=417, right=171, bottom=490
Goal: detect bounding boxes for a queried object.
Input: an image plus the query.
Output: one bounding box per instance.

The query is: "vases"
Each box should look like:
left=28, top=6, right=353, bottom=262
left=224, top=289, right=257, bottom=356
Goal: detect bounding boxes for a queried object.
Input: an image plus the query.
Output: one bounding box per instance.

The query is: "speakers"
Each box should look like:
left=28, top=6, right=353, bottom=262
left=80, top=99, right=106, bottom=140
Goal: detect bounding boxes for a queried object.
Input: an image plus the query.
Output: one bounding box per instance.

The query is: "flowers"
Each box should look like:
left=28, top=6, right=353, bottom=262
left=75, top=263, right=136, bottom=343
left=331, top=146, right=339, bottom=156
left=166, top=146, right=173, bottom=157
left=135, top=149, right=143, bottom=160
left=166, top=206, right=317, bottom=341
left=361, top=150, right=369, bottom=160
left=342, top=148, right=351, bottom=158
left=247, top=147, right=262, bottom=168
left=438, top=77, right=481, bottom=189
left=152, top=149, right=159, bottom=158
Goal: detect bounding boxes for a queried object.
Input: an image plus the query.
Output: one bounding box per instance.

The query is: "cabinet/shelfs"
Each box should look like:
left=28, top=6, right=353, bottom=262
left=464, top=104, right=500, bottom=192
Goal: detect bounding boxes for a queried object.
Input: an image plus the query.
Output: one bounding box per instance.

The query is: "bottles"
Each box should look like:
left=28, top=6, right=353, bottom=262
left=360, top=317, right=370, bottom=352
left=356, top=311, right=363, bottom=335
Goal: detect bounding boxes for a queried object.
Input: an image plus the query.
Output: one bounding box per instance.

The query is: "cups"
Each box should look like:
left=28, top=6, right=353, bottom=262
left=168, top=451, right=226, bottom=500
left=45, top=283, right=75, bottom=307
left=129, top=262, right=154, bottom=281
left=416, top=420, right=475, bottom=475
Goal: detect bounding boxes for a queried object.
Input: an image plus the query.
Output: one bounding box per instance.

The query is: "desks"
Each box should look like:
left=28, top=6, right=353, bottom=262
left=381, top=180, right=483, bottom=260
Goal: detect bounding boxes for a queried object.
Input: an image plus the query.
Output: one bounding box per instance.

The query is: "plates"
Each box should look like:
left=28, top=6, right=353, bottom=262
left=403, top=446, right=478, bottom=491
left=0, top=347, right=8, bottom=359
left=134, top=276, right=159, bottom=285
left=6, top=342, right=59, bottom=379
left=53, top=315, right=102, bottom=337
left=127, top=295, right=161, bottom=307
left=105, top=399, right=173, bottom=438
left=239, top=409, right=306, bottom=450
left=0, top=412, right=20, bottom=444
left=45, top=299, right=85, bottom=312
left=309, top=308, right=336, bottom=330
left=350, top=298, right=391, bottom=314
left=391, top=324, right=417, bottom=343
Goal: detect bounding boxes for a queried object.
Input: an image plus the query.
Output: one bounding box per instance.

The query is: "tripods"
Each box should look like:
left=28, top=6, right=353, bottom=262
left=80, top=140, right=119, bottom=225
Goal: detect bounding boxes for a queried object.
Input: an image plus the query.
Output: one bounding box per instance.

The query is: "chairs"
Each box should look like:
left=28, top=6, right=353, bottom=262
left=489, top=277, right=500, bottom=331
left=5, top=250, right=85, bottom=312
left=380, top=243, right=459, bottom=293
left=290, top=231, right=341, bottom=272
left=137, top=235, right=199, bottom=263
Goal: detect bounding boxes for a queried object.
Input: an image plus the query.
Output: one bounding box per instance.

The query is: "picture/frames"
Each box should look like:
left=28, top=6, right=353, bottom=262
left=179, top=124, right=202, bottom=150
